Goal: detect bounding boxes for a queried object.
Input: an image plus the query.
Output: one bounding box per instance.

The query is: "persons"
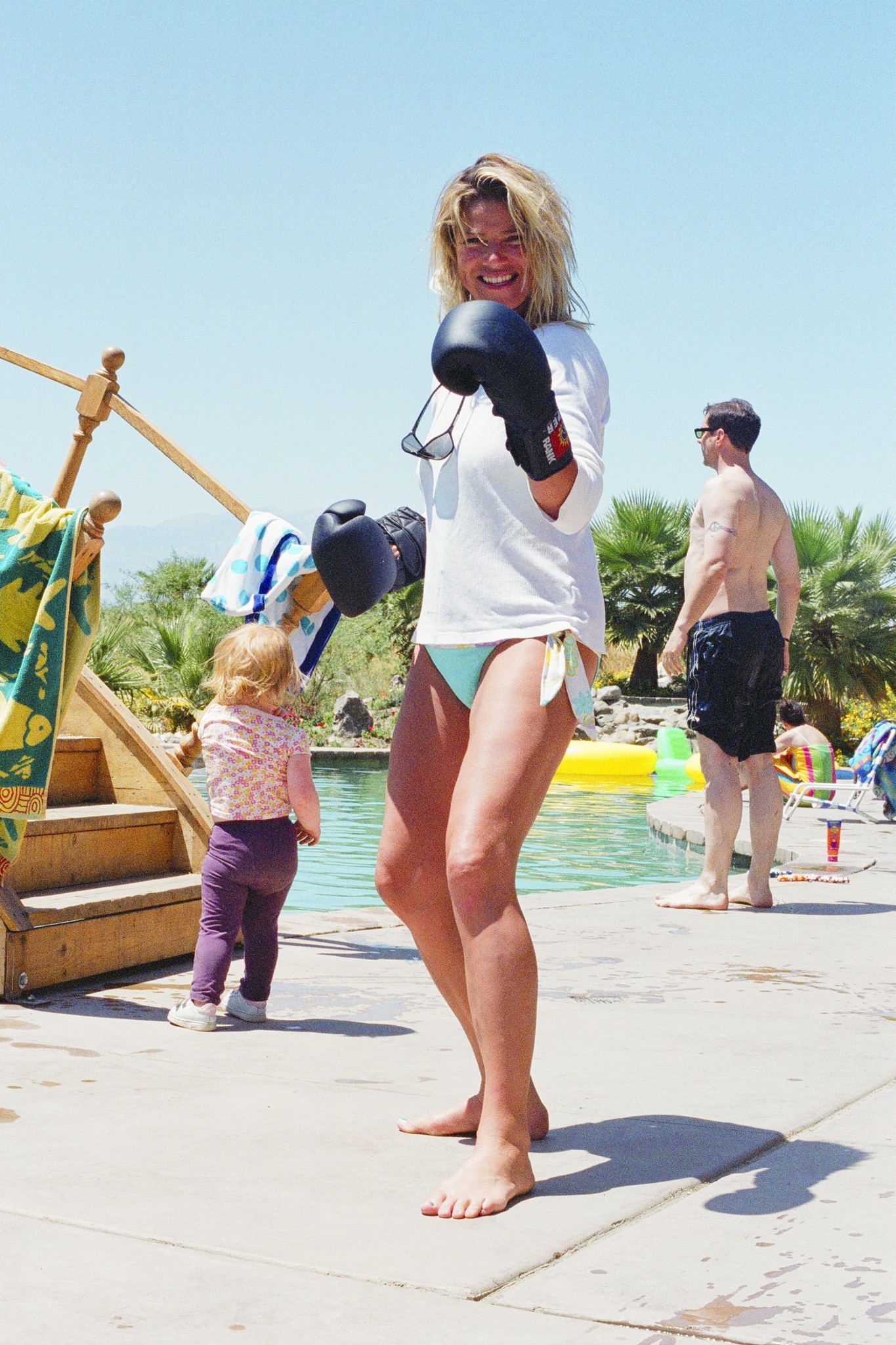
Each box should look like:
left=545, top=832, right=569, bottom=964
left=739, top=699, right=836, bottom=809
left=332, top=691, right=374, bottom=748
left=166, top=622, right=321, bottom=1032
left=654, top=399, right=802, bottom=910
left=310, top=153, right=609, bottom=1219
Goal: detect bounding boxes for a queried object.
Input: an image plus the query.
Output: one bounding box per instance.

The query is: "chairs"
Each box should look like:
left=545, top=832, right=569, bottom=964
left=783, top=722, right=896, bottom=823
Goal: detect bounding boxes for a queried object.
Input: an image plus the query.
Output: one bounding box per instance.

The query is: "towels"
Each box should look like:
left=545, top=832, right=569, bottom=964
left=846, top=718, right=896, bottom=823
left=199, top=509, right=341, bottom=698
left=0, top=464, right=100, bottom=889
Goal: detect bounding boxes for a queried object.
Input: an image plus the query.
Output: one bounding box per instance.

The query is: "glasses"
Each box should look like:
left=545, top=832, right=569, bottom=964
left=694, top=428, right=730, bottom=439
left=400, top=383, right=465, bottom=461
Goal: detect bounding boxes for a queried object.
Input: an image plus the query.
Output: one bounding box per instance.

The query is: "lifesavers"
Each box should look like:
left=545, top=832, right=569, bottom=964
left=687, top=753, right=708, bottom=783
left=555, top=737, right=658, bottom=776
left=654, top=726, right=689, bottom=778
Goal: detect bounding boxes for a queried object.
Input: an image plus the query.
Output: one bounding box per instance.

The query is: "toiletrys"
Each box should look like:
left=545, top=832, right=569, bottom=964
left=826, top=818, right=841, bottom=862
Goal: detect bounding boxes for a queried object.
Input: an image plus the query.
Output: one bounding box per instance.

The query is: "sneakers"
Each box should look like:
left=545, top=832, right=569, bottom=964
left=221, top=988, right=266, bottom=1023
left=167, top=998, right=217, bottom=1031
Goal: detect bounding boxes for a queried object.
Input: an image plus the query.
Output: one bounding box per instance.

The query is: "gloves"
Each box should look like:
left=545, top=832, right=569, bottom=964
left=312, top=500, right=427, bottom=618
left=436, top=302, right=574, bottom=481
